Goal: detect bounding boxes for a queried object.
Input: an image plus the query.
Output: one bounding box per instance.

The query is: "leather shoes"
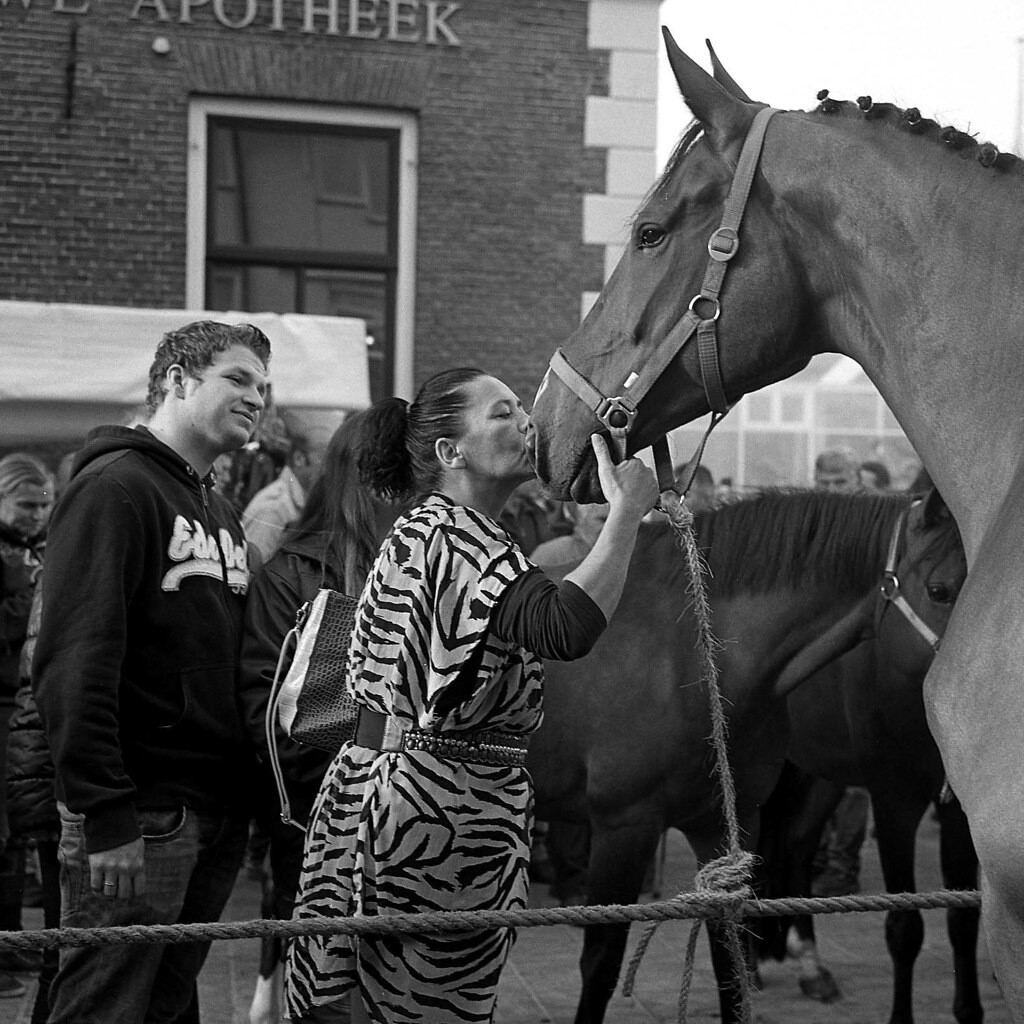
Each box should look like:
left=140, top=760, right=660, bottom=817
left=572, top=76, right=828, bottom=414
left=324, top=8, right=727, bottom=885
left=3, top=950, right=43, bottom=978
left=0, top=973, right=27, bottom=999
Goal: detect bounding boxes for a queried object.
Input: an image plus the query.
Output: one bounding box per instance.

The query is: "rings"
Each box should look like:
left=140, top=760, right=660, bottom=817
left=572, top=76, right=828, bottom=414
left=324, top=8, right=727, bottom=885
left=102, top=879, right=116, bottom=888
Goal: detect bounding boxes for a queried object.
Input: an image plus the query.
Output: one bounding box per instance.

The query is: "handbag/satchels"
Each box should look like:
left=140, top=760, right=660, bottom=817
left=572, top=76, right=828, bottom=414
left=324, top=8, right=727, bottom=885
left=277, top=536, right=360, bottom=758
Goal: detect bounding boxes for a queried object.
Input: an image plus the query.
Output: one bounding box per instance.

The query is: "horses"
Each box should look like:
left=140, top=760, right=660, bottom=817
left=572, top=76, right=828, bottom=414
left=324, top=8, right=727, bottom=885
left=240, top=22, right=1023, bottom=1024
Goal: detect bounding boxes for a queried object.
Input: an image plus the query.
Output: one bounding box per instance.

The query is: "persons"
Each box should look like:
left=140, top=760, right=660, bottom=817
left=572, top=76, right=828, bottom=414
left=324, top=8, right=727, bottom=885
left=29, top=318, right=275, bottom=1023
left=279, top=364, right=663, bottom=1023
left=677, top=460, right=715, bottom=517
left=0, top=452, right=56, bottom=993
left=814, top=454, right=920, bottom=897
left=235, top=422, right=334, bottom=569
left=4, top=571, right=62, bottom=1024
left=243, top=406, right=398, bottom=883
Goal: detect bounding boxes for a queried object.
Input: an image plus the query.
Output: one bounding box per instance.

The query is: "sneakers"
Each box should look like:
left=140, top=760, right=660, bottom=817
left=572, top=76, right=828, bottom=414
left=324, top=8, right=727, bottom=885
left=812, top=875, right=859, bottom=897
